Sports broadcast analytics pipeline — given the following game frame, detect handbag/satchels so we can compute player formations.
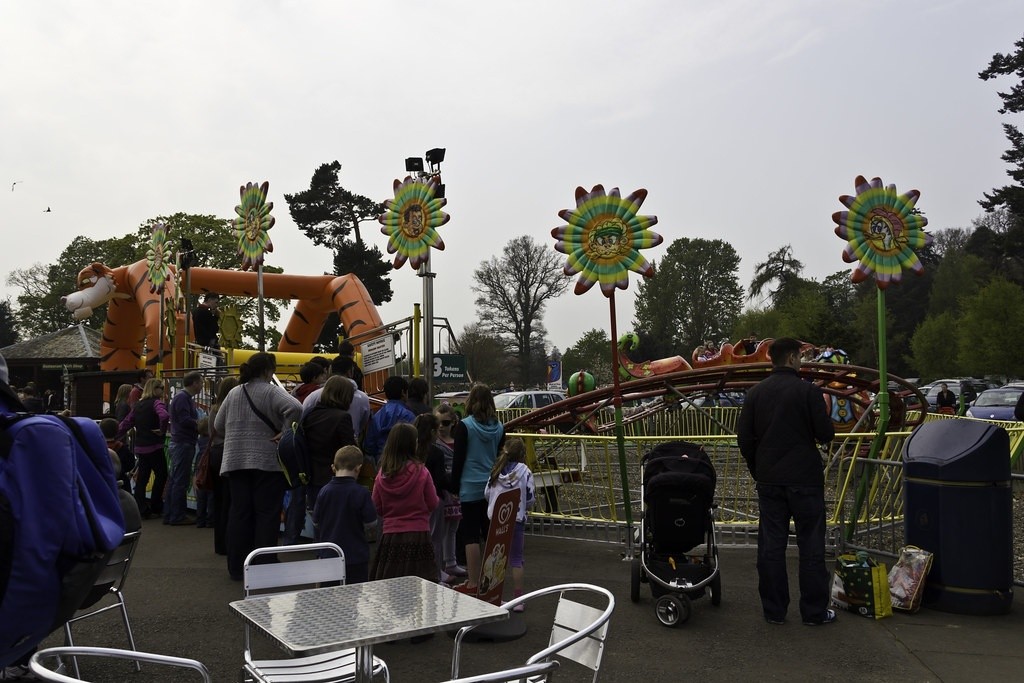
[832,554,893,619]
[886,545,934,613]
[194,445,209,491]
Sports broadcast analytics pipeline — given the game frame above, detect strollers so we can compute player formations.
[631,442,722,628]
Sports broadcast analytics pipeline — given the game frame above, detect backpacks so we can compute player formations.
[0,416,126,665]
[276,407,310,488]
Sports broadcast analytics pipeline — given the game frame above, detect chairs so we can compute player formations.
[233,542,393,683]
[451,581,618,683]
[27,645,214,683]
[62,526,144,679]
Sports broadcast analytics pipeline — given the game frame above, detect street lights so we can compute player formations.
[404,147,448,411]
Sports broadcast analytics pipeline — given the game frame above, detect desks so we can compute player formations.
[227,574,512,683]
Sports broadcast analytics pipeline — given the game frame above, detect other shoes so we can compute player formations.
[804,610,835,626]
[445,564,466,575]
[441,570,456,582]
[141,512,159,519]
[411,633,434,644]
[170,515,195,525]
[453,580,480,596]
[384,641,397,644]
[765,614,785,625]
[512,604,523,612]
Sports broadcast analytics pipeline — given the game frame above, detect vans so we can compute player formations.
[493,391,569,418]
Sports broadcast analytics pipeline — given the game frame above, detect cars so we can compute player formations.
[889,377,1024,421]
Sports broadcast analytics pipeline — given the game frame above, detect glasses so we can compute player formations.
[155,386,164,390]
[441,420,457,427]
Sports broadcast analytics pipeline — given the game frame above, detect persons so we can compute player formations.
[0,340,537,680]
[738,337,837,626]
[697,331,833,384]
[193,291,225,373]
[1014,391,1024,422]
[935,383,956,410]
